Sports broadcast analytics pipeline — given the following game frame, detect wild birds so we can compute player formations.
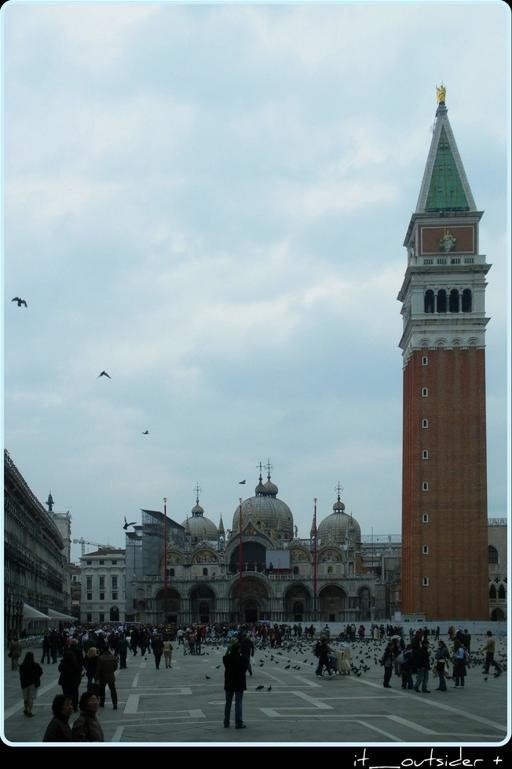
[99,370,111,379]
[142,430,149,434]
[238,480,246,484]
[255,685,264,691]
[200,638,507,682]
[11,296,28,308]
[267,685,272,691]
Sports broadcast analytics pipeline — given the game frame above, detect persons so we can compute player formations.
[6,621,503,742]
